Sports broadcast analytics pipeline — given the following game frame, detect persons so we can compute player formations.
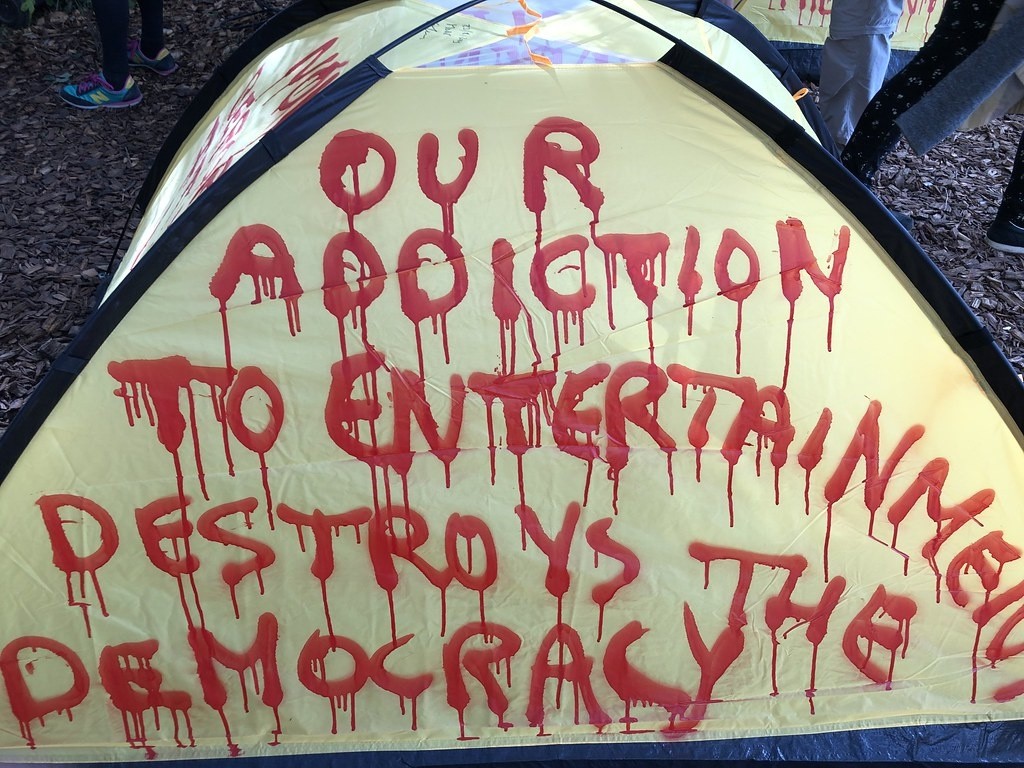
[58,1,181,110]
[834,2,1024,254]
[815,1,903,169]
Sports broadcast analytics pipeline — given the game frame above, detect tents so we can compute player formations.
[706,0,1011,136]
[0,0,1024,768]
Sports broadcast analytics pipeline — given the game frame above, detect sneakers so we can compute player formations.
[984,218,1024,256]
[126,42,179,76]
[60,70,144,109]
